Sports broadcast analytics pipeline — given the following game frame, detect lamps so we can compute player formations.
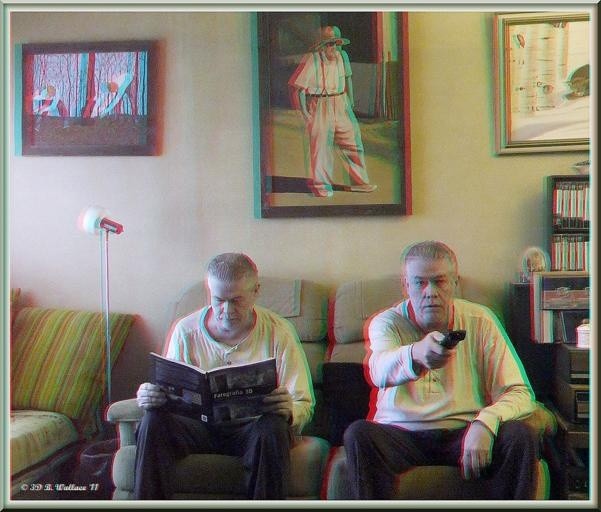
[82,204,130,408]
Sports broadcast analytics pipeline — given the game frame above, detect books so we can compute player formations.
[552,233,587,271]
[554,182,588,227]
[542,309,577,342]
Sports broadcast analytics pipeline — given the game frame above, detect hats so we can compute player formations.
[309,25,350,52]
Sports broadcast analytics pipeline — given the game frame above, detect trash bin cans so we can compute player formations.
[80,440,119,496]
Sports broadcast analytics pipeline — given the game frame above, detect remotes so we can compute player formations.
[438,330,468,350]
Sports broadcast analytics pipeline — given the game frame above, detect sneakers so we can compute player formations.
[344,185,378,192]
[315,191,335,197]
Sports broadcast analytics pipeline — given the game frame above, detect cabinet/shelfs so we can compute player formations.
[554,343,589,424]
[543,175,591,273]
[529,268,591,347]
[549,402,591,501]
[508,279,554,410]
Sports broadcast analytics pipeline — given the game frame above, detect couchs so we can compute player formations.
[321,270,556,501]
[104,275,332,499]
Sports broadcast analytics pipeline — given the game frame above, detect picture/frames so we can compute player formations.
[21,38,162,157]
[254,12,416,220]
[490,12,592,157]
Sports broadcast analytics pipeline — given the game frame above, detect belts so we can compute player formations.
[307,92,344,97]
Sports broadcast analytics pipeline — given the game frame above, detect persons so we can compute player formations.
[129,252,316,500]
[344,241,538,500]
[293,26,378,198]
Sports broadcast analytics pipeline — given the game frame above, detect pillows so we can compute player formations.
[9,286,21,324]
[10,304,139,422]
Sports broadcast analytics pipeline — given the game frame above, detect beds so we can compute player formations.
[9,294,143,499]
